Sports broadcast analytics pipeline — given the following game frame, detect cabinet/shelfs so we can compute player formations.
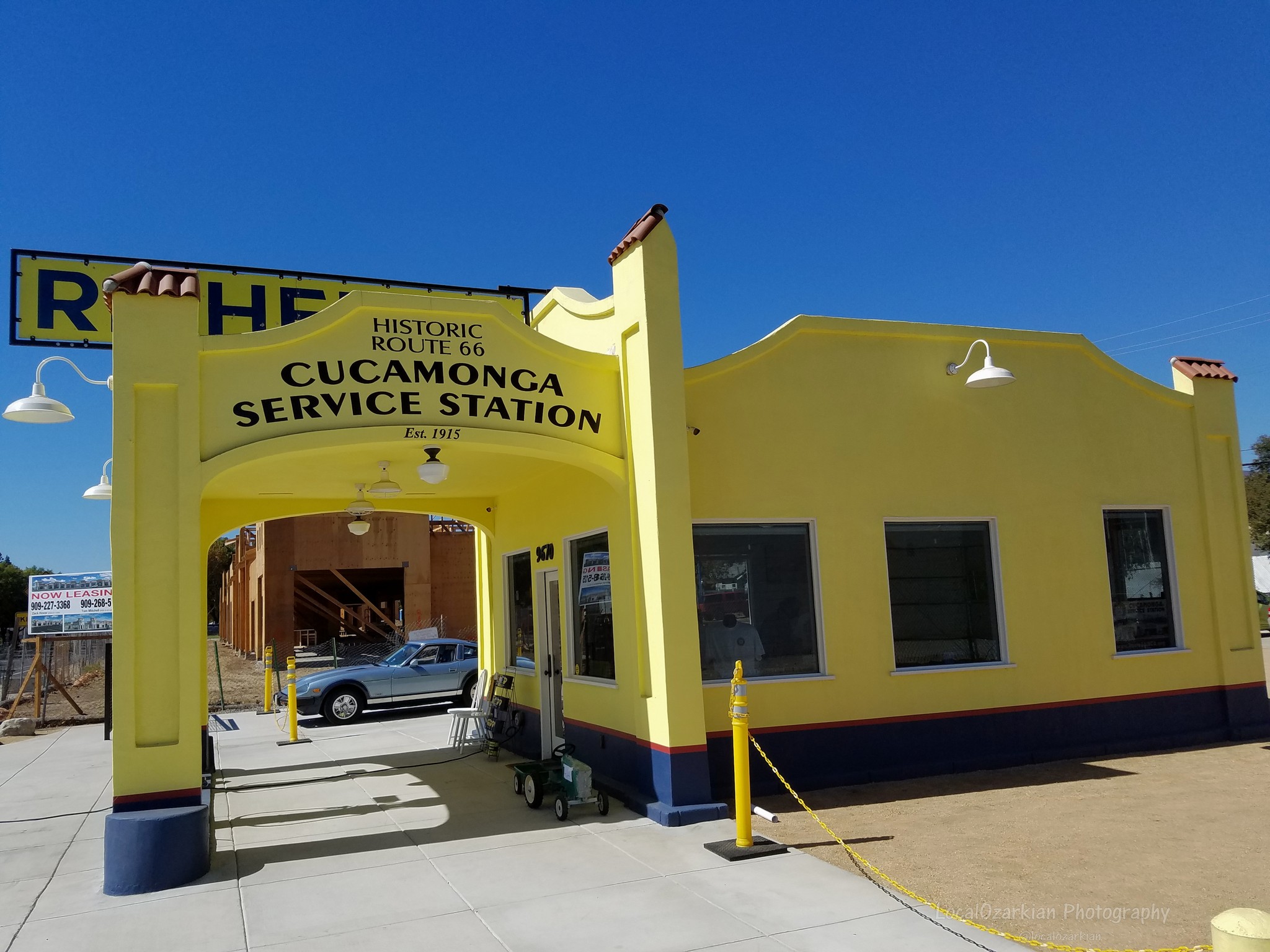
[479,665,516,762]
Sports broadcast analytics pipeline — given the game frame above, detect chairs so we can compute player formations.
[447,669,497,755]
[438,649,455,662]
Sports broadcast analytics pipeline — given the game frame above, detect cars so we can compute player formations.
[1250,543,1270,631]
[274,638,536,726]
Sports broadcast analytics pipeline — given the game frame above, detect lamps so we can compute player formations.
[1,356,114,424]
[416,443,449,485]
[82,459,112,501]
[947,340,1017,389]
[366,461,403,499]
[344,482,375,517]
[347,512,371,536]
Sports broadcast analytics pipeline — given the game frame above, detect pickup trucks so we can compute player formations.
[207,623,219,635]
